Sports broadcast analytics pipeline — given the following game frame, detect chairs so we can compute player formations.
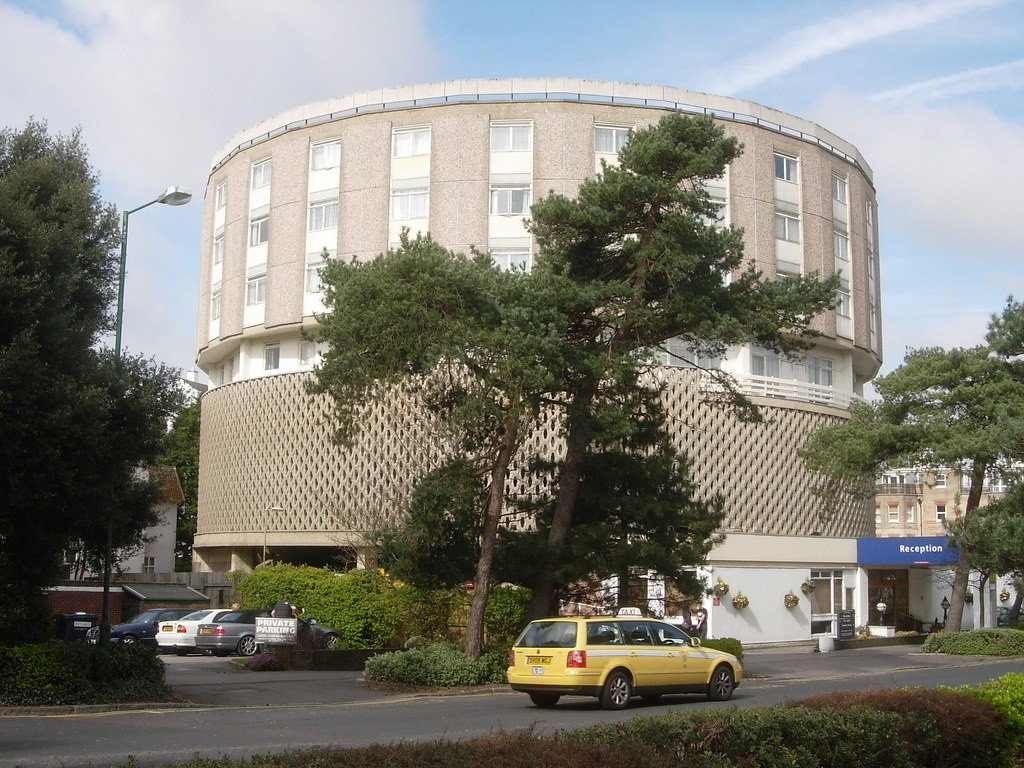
[633,629,650,644]
[602,631,615,644]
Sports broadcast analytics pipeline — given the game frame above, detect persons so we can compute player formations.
[271,597,296,618]
[696,602,707,637]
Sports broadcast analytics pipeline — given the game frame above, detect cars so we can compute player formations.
[194,608,342,656]
[86,608,203,655]
[154,608,235,656]
[506,607,743,710]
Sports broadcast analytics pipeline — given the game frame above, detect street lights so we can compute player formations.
[263,507,284,567]
[98,186,193,647]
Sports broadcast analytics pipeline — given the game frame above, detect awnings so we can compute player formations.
[123,582,210,602]
